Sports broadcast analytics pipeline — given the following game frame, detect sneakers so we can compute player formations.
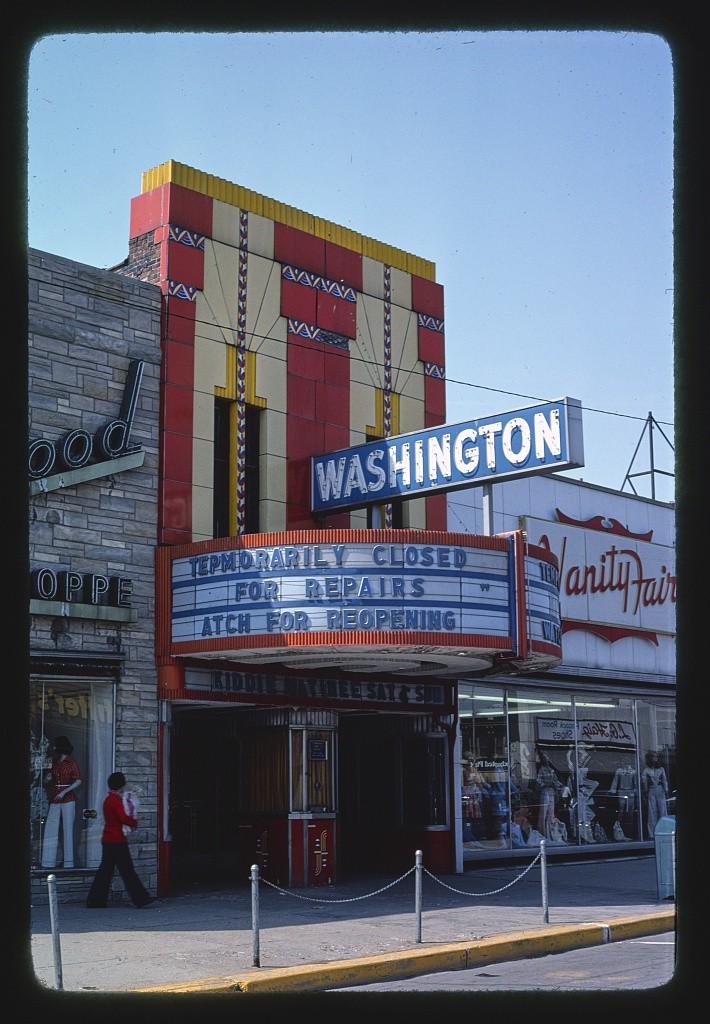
[85,901,110,908]
[137,899,162,908]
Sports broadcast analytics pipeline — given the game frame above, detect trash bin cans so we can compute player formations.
[654,814,676,901]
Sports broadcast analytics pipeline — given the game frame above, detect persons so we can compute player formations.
[39,736,83,870]
[609,753,646,842]
[85,771,161,909]
[534,750,570,839]
[461,750,491,842]
[640,750,671,839]
[487,760,522,839]
